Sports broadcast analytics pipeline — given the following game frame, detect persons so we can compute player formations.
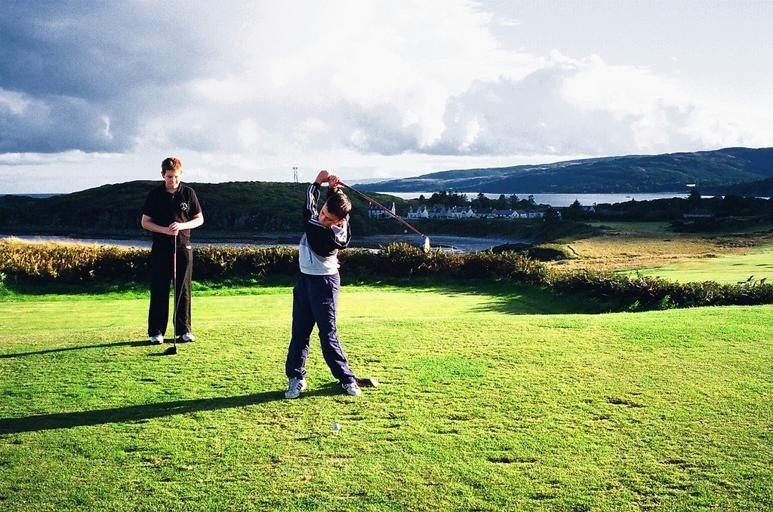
[139,156,205,345]
[282,170,366,399]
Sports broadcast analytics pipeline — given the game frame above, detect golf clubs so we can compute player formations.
[163,235,177,354]
[327,176,431,254]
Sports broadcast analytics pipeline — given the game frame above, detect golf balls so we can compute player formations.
[333,423,340,430]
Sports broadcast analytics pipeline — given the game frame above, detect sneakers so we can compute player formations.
[285,377,307,399]
[342,382,362,396]
[151,334,164,343]
[182,333,195,342]
